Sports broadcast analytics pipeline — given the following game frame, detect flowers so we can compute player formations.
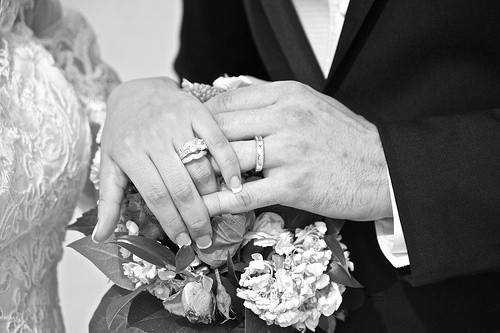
[65,177,363,333]
